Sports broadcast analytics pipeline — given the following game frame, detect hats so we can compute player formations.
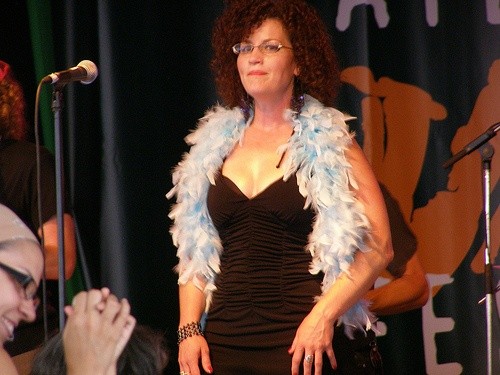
[0,202,40,246]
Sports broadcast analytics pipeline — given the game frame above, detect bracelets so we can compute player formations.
[176,322,205,345]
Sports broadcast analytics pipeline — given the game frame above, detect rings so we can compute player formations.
[304,354,313,363]
[180,371,190,375]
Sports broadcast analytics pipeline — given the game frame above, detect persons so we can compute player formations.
[165,0,395,375]
[363,174,430,315]
[0,60,171,375]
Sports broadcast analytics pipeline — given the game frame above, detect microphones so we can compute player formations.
[42,60,98,84]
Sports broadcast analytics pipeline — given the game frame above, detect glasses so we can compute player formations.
[0,261,40,308]
[232,40,292,56]
